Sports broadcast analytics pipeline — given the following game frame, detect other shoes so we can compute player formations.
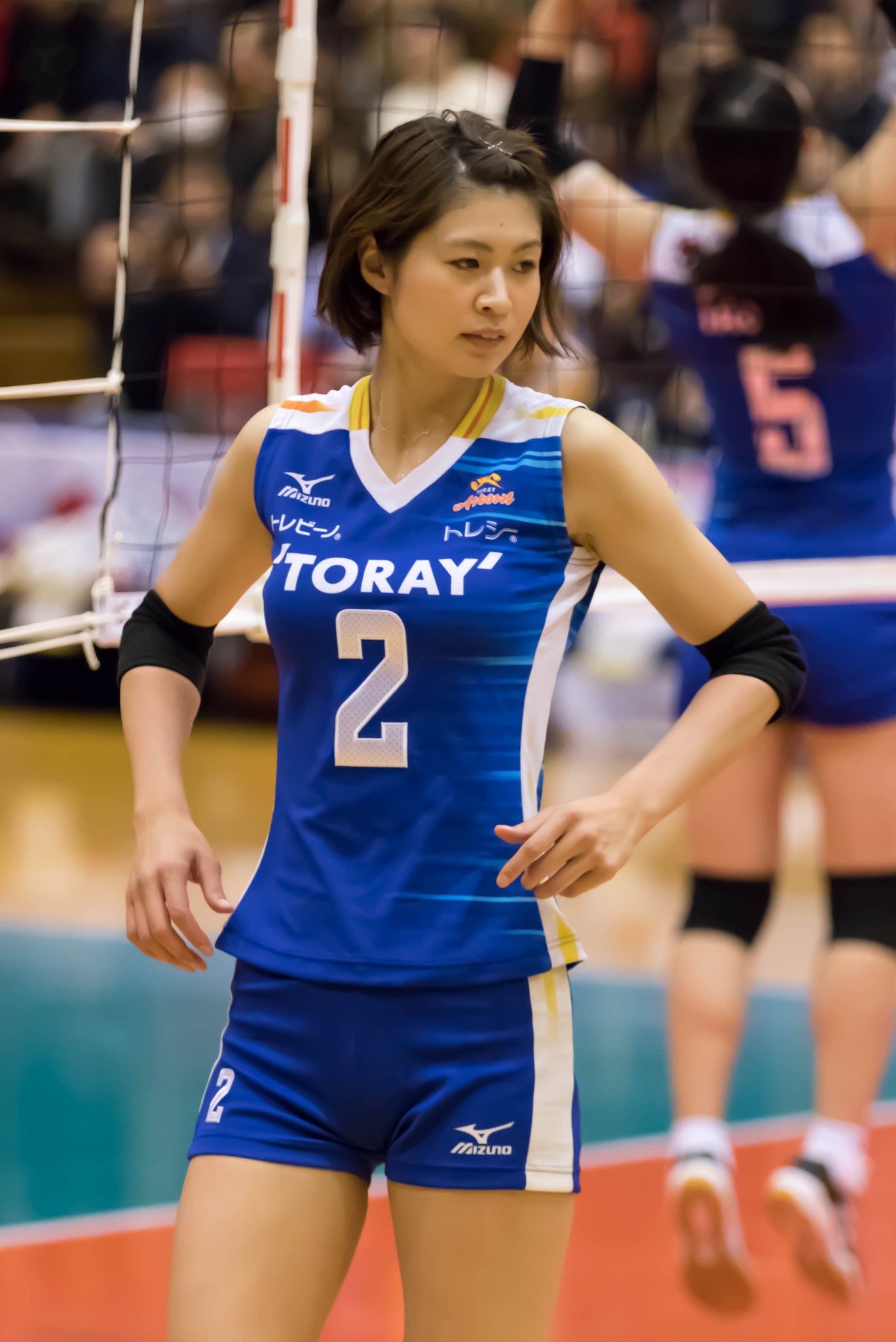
[763,1156,861,1303]
[665,1151,756,1318]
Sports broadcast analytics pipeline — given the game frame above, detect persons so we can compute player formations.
[0,1,894,1317]
[114,112,796,1342]
